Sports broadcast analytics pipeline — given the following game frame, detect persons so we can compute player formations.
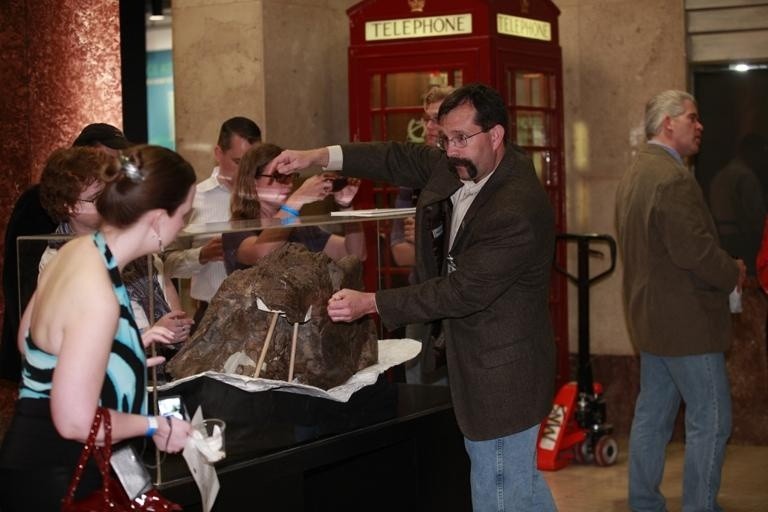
[38,142,197,385]
[755,214,768,296]
[709,133,765,254]
[0,122,129,383]
[612,88,748,512]
[275,30,561,511]
[164,115,263,336]
[388,84,458,385]
[221,140,370,275]
[0,145,196,512]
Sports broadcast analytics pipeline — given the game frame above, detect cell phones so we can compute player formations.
[157,395,183,419]
[326,176,349,192]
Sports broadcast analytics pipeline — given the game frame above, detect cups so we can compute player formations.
[192,418,227,464]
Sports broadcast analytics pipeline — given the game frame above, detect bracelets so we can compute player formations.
[279,204,300,217]
[145,414,159,436]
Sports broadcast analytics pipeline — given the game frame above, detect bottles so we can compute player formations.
[728,285,743,314]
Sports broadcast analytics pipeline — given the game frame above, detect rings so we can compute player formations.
[178,320,182,326]
[181,325,185,331]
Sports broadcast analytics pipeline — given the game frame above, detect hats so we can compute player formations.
[81,123,129,149]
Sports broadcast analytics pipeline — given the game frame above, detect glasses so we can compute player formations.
[434,131,485,150]
[76,192,98,206]
[261,171,296,185]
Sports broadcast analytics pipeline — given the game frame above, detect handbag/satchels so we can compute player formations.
[63,407,182,510]
